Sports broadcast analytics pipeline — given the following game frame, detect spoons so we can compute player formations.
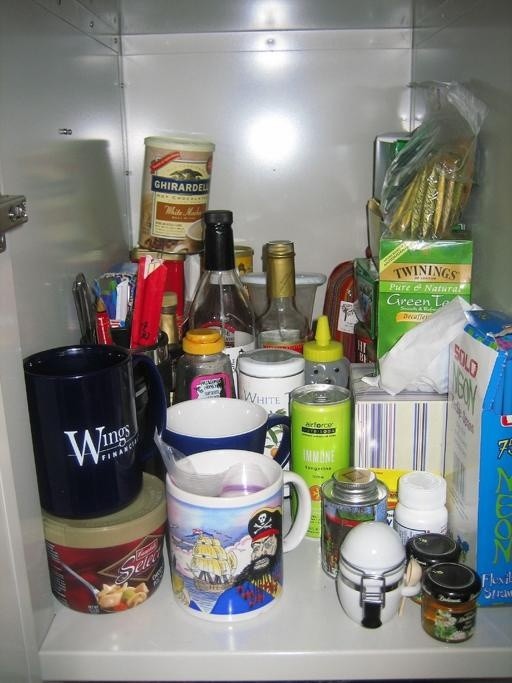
[396,557,422,617]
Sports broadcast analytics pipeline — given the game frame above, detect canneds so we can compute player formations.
[406,533,460,605]
[420,563,481,643]
[320,466,388,580]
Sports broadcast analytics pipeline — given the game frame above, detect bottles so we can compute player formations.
[190,210,254,332]
[289,383,351,540]
[303,315,350,389]
[138,136,216,255]
[393,470,448,547]
[258,241,310,349]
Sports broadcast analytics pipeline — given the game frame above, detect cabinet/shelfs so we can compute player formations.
[0,1,512,682]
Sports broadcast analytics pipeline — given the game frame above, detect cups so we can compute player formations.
[23,345,167,519]
[240,271,328,329]
[166,399,291,470]
[166,449,312,622]
